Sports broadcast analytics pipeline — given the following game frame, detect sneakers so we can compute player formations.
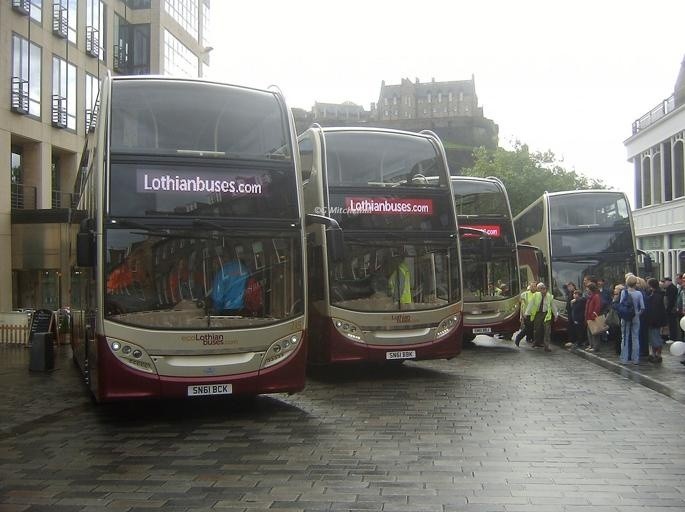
[526,337,532,343]
[618,358,628,365]
[544,348,552,352]
[565,340,590,349]
[654,356,663,363]
[648,355,658,364]
[632,361,640,367]
[515,336,520,347]
[665,339,677,344]
[589,347,600,352]
[534,342,545,348]
[584,347,595,352]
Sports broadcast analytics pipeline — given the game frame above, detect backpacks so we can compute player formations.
[242,270,265,311]
[615,290,636,321]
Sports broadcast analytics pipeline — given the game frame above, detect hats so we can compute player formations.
[661,277,672,282]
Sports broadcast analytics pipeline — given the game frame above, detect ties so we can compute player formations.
[538,295,546,312]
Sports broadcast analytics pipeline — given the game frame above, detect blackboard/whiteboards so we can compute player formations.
[27,309,53,344]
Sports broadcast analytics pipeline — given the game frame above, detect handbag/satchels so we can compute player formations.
[570,319,586,340]
[604,308,621,328]
[586,312,610,336]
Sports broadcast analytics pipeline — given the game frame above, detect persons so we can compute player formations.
[515,269,685,364]
